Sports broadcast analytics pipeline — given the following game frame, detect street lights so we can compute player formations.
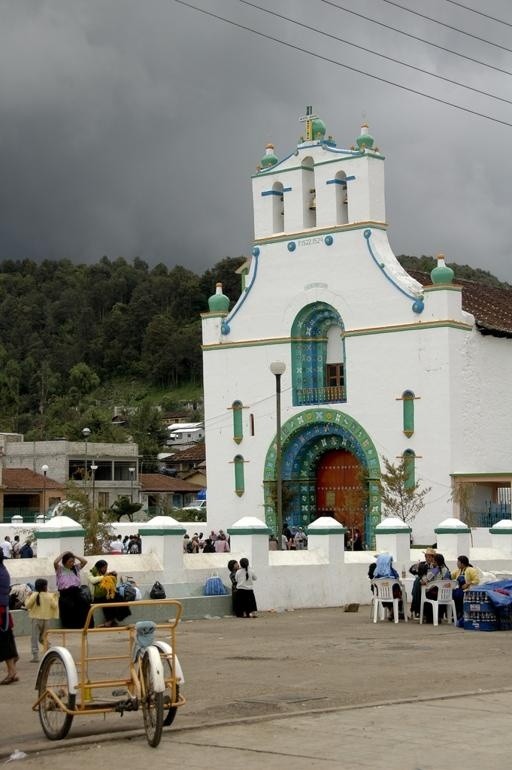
[268,359,287,552]
[36,426,136,536]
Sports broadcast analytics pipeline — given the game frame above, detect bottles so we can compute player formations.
[401,563,406,578]
[467,592,512,624]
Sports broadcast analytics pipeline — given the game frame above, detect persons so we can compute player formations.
[88,560,131,625]
[229,559,259,617]
[343,528,362,550]
[367,548,478,624]
[184,530,230,553]
[106,533,142,555]
[277,522,307,551]
[0,524,34,560]
[24,579,59,663]
[0,549,20,682]
[53,551,95,629]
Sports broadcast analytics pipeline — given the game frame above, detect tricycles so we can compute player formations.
[32,597,185,751]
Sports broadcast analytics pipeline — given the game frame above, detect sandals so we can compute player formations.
[1,673,18,684]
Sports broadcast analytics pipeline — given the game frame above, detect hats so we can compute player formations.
[422,548,436,555]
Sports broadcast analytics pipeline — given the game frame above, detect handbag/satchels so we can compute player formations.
[118,577,142,601]
[204,571,228,595]
[150,581,166,599]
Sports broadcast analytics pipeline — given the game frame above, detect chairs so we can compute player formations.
[371,577,410,623]
[419,580,458,626]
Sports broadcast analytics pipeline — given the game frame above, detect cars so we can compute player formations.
[181,499,206,510]
